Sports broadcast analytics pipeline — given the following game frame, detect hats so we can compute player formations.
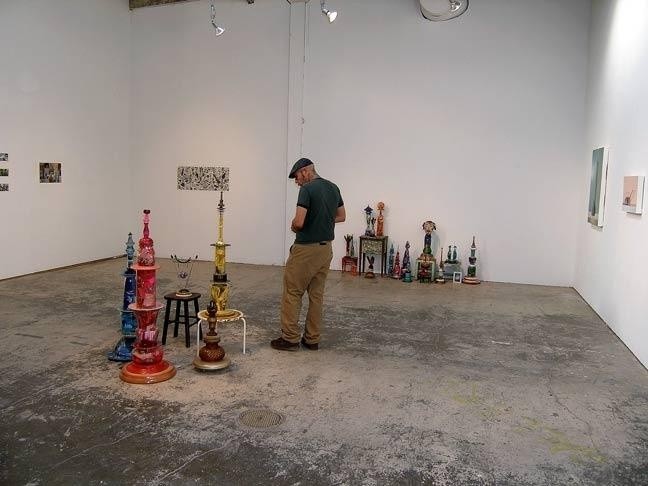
[288,158,313,179]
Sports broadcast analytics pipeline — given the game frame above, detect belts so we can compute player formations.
[320,243,326,245]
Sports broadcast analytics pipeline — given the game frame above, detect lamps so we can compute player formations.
[319,1,339,22]
[205,0,226,39]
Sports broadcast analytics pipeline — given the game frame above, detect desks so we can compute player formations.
[358,234,389,279]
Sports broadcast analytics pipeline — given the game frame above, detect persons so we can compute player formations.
[269,157,347,353]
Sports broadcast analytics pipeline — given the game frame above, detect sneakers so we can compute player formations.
[270,336,318,351]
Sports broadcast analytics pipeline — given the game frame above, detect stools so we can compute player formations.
[161,290,206,348]
[341,256,357,276]
[194,307,247,359]
[415,259,435,282]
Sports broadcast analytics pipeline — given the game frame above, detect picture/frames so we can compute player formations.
[586,146,609,230]
[619,173,644,216]
[452,271,462,284]
